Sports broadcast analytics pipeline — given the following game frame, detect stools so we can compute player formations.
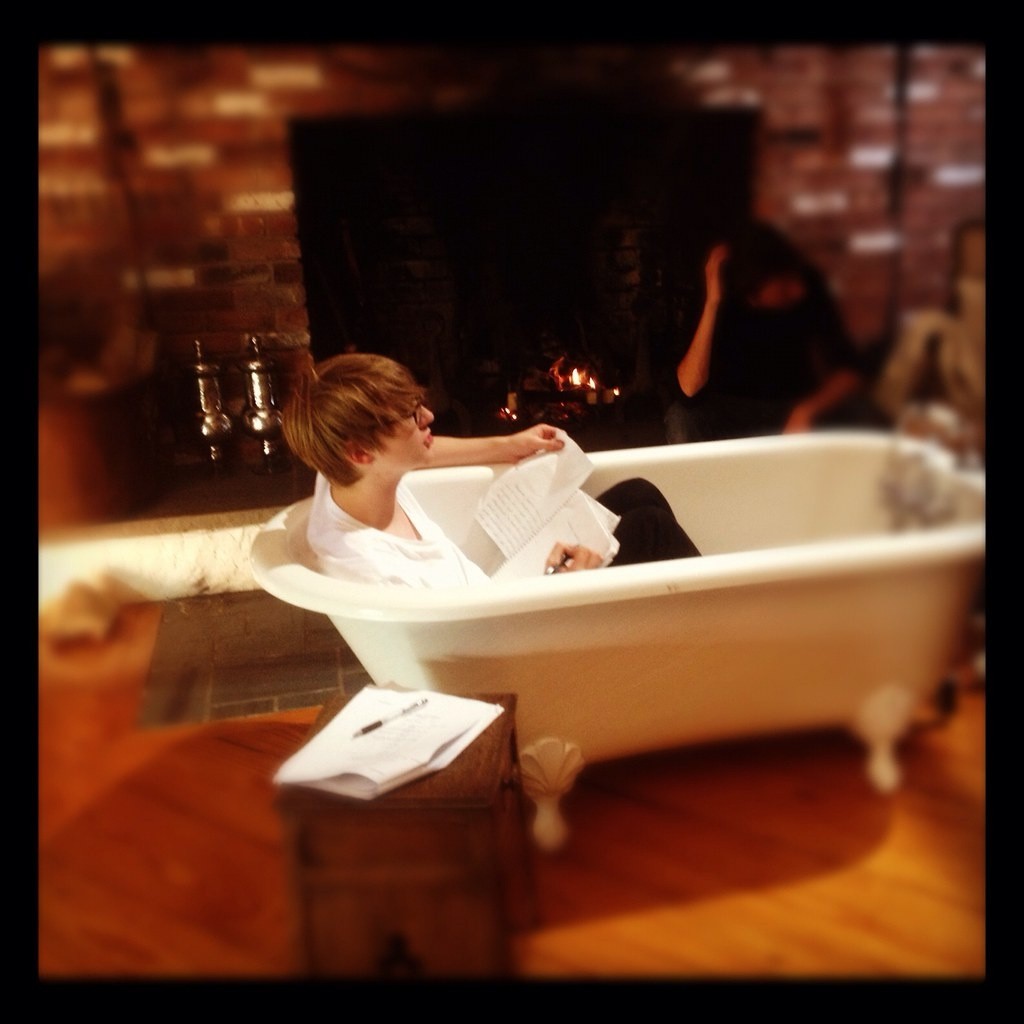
[292,688,532,981]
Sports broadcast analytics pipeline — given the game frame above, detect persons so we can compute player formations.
[281,355,700,588]
[649,219,985,470]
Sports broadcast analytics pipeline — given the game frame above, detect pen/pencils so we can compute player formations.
[545,543,581,575]
[353,698,428,739]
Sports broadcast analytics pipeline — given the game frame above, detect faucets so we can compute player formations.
[903,401,985,472]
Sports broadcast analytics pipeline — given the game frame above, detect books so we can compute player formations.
[488,488,622,581]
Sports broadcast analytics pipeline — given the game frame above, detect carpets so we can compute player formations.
[137,586,344,735]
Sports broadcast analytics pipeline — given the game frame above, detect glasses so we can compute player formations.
[369,399,423,430]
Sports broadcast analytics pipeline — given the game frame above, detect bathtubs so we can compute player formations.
[242,426,985,793]
